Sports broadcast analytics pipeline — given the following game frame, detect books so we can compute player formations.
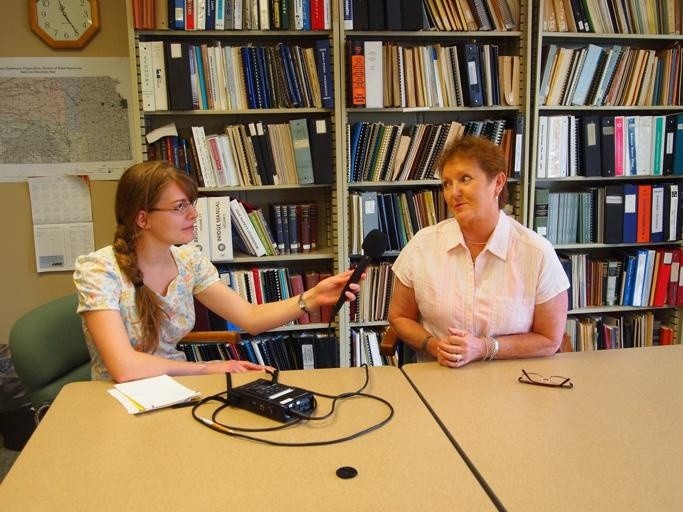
[350,185,515,255]
[349,260,394,323]
[351,327,437,368]
[132,1,337,371]
[533,1,682,353]
[345,35,520,108]
[108,374,203,415]
[346,117,524,183]
[343,0,521,32]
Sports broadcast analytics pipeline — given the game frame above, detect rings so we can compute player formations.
[456,353,464,362]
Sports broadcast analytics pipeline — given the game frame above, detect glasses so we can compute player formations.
[147,199,196,214]
[519,369,573,388]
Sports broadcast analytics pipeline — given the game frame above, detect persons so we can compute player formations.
[73,159,368,384]
[389,134,571,368]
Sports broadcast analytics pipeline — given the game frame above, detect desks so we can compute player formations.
[402,335,680,511]
[2,359,505,511]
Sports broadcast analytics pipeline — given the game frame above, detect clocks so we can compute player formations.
[26,0,103,50]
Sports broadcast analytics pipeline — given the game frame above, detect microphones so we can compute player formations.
[328,229,388,319]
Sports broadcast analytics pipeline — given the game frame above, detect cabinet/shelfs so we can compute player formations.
[335,0,534,370]
[121,0,344,374]
[533,1,681,353]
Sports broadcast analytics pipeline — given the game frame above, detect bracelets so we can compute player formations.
[481,336,499,361]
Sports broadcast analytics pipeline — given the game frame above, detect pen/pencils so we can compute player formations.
[172,400,199,409]
[201,418,232,434]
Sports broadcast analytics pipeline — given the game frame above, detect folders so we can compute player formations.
[653,248,683,305]
[464,43,483,107]
[164,41,194,111]
[289,112,334,185]
[362,191,379,241]
[605,185,637,244]
[581,115,615,178]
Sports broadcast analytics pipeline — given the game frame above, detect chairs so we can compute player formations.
[8,292,240,428]
[377,313,571,366]
